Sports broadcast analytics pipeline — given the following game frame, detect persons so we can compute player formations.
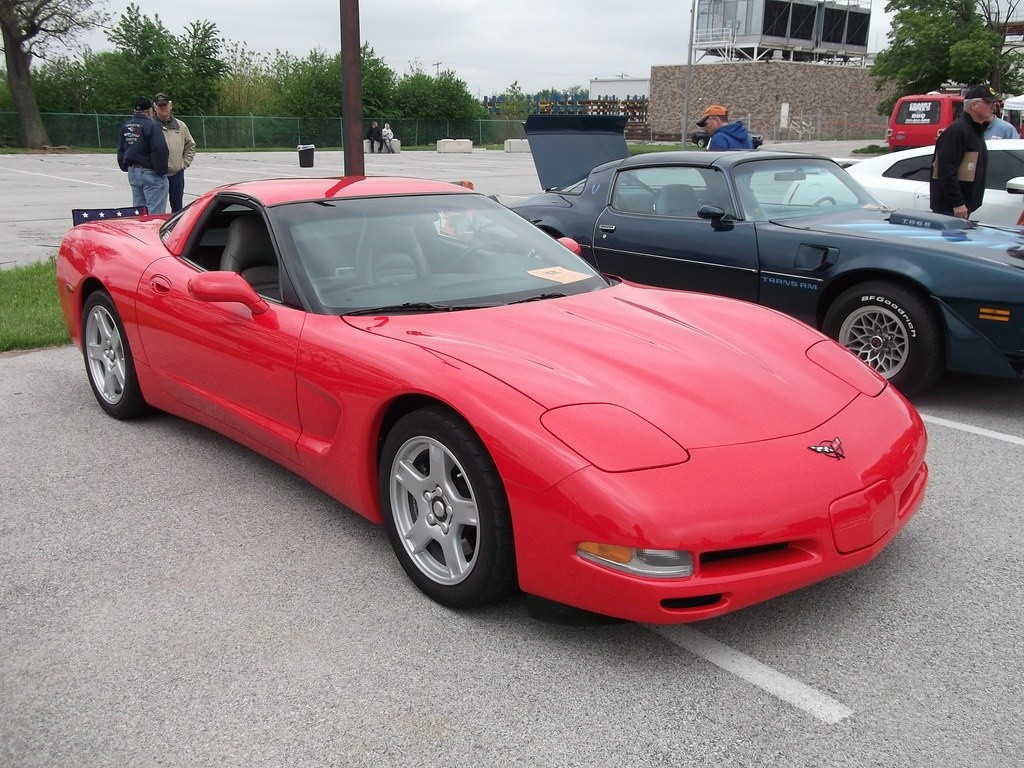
[117,96,169,214]
[696,105,753,185]
[929,85,1002,219]
[382,123,395,153]
[983,114,1018,140]
[367,122,384,152]
[150,94,196,213]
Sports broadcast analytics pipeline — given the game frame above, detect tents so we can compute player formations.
[1001,94,1024,120]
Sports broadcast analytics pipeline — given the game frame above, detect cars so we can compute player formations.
[782,139,1024,239]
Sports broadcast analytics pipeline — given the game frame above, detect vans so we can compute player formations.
[886,88,964,152]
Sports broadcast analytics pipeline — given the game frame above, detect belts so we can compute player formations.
[130,163,145,168]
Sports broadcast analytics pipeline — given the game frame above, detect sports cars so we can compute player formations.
[55,175,929,627]
[496,151,1024,401]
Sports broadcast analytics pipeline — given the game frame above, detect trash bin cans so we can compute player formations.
[298,145,315,168]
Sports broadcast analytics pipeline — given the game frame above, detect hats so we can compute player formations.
[964,84,1002,103]
[154,93,169,106]
[134,97,154,112]
[696,105,728,127]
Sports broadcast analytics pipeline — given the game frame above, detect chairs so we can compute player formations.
[219,212,281,299]
[356,222,431,285]
[720,183,766,217]
[651,183,699,216]
